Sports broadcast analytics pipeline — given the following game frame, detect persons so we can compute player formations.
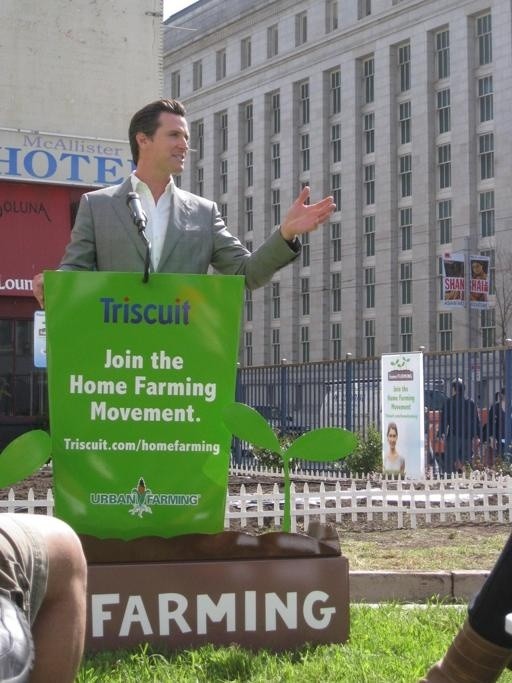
[33,98,336,310]
[472,261,487,278]
[384,422,405,477]
[436,378,482,475]
[1,512,88,682]
[487,387,505,448]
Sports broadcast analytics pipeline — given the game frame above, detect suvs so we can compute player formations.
[240,399,313,460]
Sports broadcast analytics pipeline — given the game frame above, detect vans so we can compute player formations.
[317,375,494,468]
